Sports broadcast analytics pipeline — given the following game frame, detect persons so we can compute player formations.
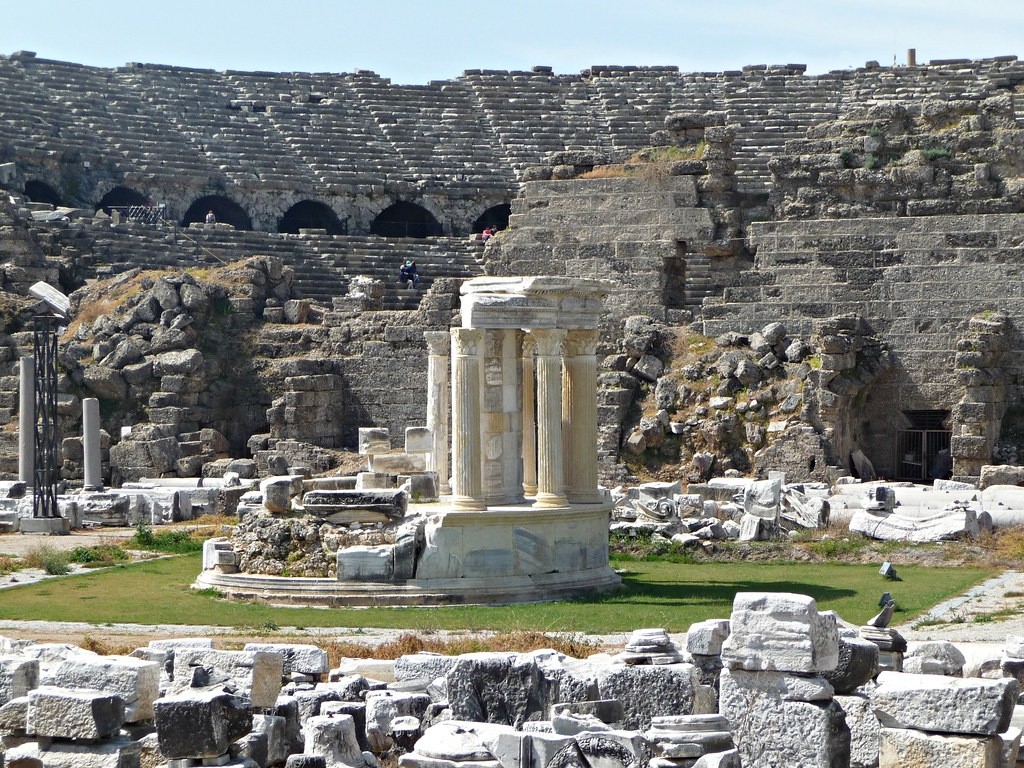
[206,210,216,223]
[489,224,498,236]
[398,257,422,292]
[482,227,492,241]
